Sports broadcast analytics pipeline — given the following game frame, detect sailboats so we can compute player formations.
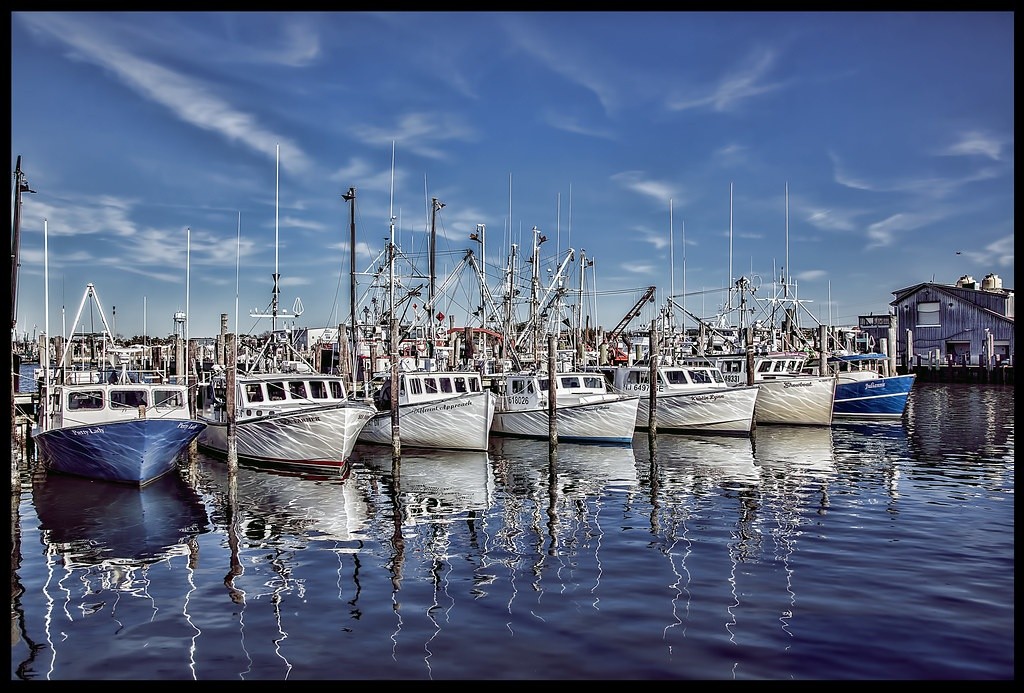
[31,462,213,680]
[26,138,918,487]
[26,214,208,491]
[193,413,917,660]
